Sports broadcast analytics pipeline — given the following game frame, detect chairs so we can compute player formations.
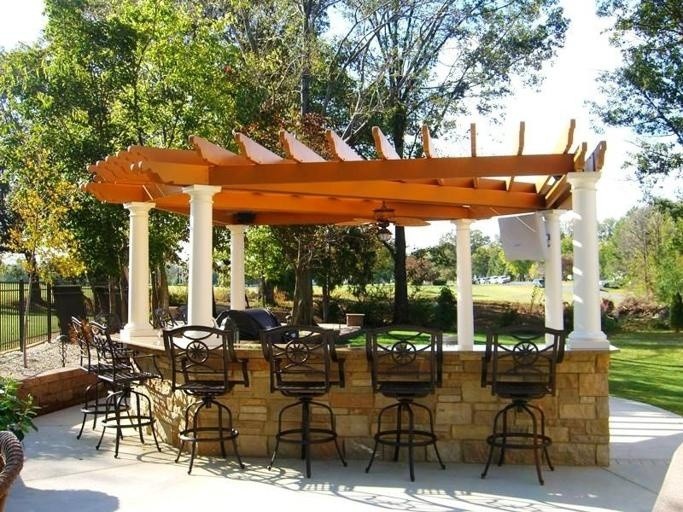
[0,430,24,512]
[154,307,178,328]
[259,322,347,477]
[69,315,122,438]
[362,317,444,481]
[162,325,248,475]
[477,318,566,485]
[85,320,161,459]
